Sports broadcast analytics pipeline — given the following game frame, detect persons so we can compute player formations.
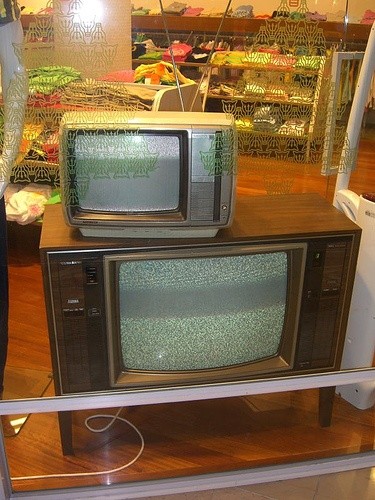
[0,1,29,400]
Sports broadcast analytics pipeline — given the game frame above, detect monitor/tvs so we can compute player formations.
[37,190,363,456]
[58,110,238,238]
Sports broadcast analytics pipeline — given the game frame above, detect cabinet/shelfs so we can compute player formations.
[0,84,204,228]
[132,54,331,159]
